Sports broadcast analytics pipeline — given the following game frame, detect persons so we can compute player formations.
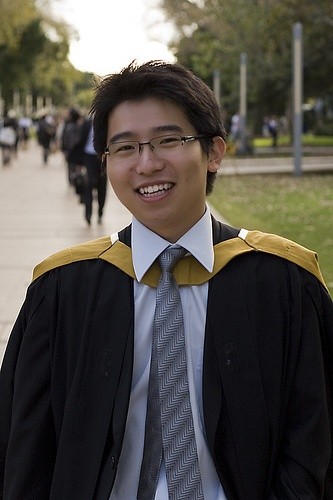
[0,98,332,226]
[0,57,333,500]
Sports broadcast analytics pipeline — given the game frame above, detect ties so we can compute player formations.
[137,246,207,500]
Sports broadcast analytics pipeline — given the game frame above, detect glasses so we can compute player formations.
[102,134,205,162]
